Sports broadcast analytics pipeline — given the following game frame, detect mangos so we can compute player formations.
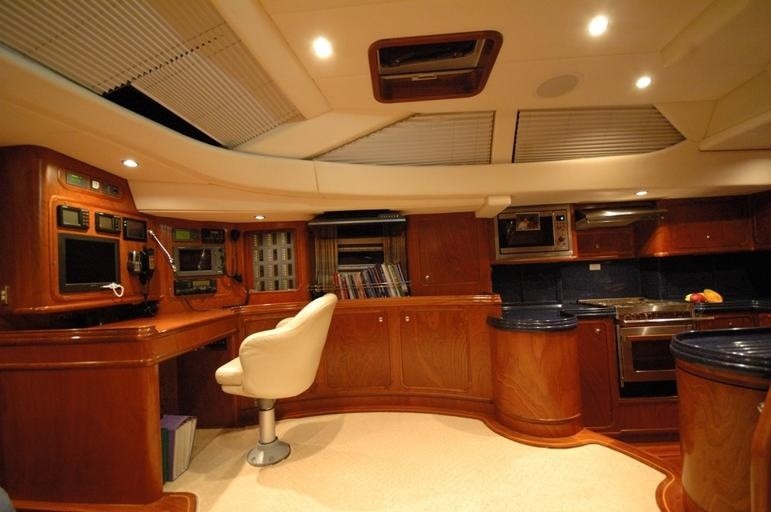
[703,289,723,303]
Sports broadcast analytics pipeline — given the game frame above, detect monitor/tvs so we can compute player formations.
[57,233,120,292]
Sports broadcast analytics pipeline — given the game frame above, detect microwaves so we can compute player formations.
[496,204,575,261]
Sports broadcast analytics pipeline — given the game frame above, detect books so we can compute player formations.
[335,263,409,299]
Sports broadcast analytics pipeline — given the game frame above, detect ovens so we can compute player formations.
[618,312,692,397]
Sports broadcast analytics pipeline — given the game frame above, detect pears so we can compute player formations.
[684,294,692,302]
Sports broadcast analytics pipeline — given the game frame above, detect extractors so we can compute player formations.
[575,207,668,227]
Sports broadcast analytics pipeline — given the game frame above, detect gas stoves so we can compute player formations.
[577,297,689,310]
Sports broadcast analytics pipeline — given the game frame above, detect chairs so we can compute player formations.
[214,293,340,468]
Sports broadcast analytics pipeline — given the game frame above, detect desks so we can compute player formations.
[2,305,242,511]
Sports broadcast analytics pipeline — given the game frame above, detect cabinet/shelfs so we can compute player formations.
[659,199,755,254]
[322,297,502,420]
[691,316,756,332]
[236,314,321,421]
[577,226,635,260]
[406,212,494,295]
[615,322,696,443]
[575,318,619,437]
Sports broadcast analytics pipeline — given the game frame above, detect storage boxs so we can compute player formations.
[161,412,198,482]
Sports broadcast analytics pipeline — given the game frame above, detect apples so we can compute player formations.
[691,294,706,303]
